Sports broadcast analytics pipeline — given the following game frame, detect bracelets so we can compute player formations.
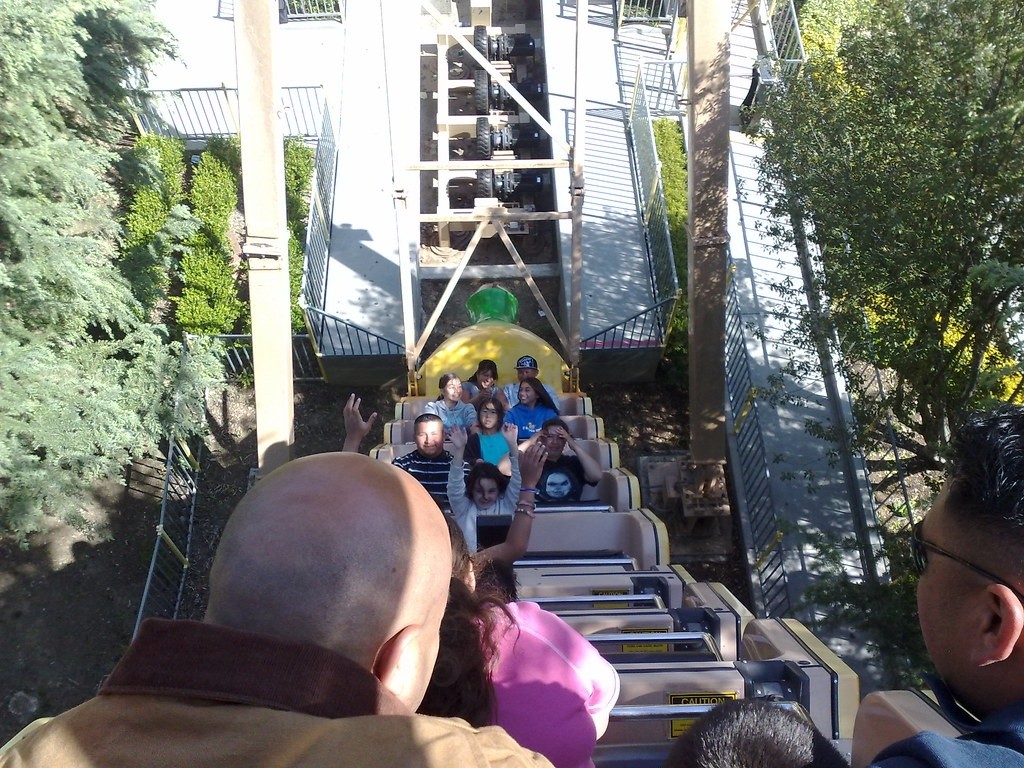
[515,508,534,518]
[519,488,540,494]
[516,501,536,509]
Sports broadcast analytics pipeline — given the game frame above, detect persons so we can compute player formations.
[866,415,1024,768]
[503,355,560,409]
[662,698,852,768]
[342,394,621,768]
[424,373,479,439]
[461,359,509,411]
[0,451,557,768]
[503,378,560,440]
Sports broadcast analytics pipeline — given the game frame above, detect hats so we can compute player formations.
[514,355,537,370]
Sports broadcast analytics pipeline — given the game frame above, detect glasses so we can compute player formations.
[912,520,1024,605]
